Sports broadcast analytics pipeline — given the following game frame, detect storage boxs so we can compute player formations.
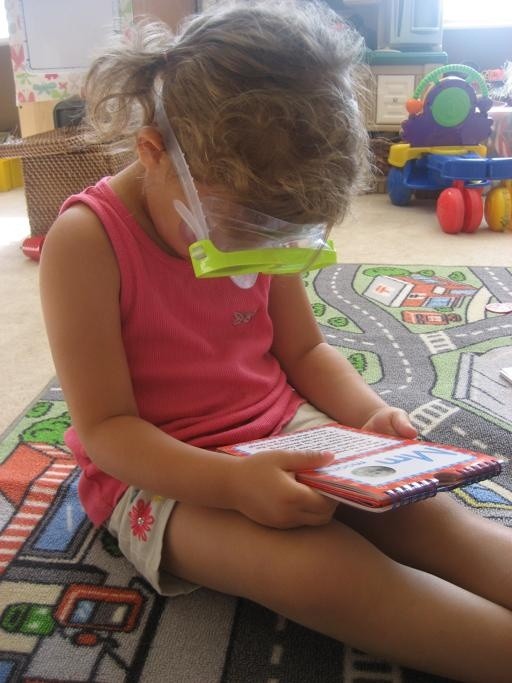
[2,124,140,239]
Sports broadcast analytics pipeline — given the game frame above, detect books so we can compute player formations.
[213,420,498,516]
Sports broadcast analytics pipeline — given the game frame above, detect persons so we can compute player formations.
[38,0,512,682]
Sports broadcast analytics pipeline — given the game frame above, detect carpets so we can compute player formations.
[0,262,509,679]
